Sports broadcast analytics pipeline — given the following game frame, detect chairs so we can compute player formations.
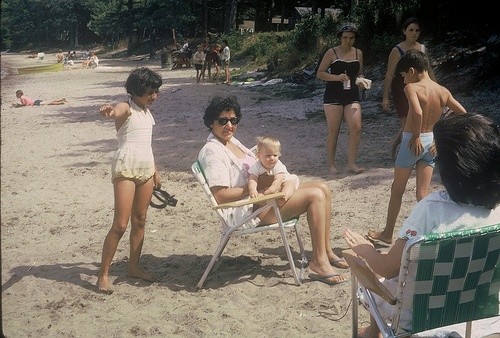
[337,225,499,337]
[192,160,308,292]
[171,54,191,70]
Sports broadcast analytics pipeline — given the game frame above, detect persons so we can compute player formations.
[315,21,364,174]
[382,20,435,160]
[197,93,353,286]
[249,135,302,208]
[22,43,106,70]
[340,108,500,338]
[13,90,66,107]
[96,68,163,296]
[160,23,240,84]
[365,49,469,244]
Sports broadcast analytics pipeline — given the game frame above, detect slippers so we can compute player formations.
[309,273,347,284]
[330,257,350,269]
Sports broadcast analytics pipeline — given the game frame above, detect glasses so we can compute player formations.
[341,25,358,32]
[215,118,240,126]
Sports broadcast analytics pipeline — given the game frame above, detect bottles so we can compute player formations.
[343,80,351,90]
